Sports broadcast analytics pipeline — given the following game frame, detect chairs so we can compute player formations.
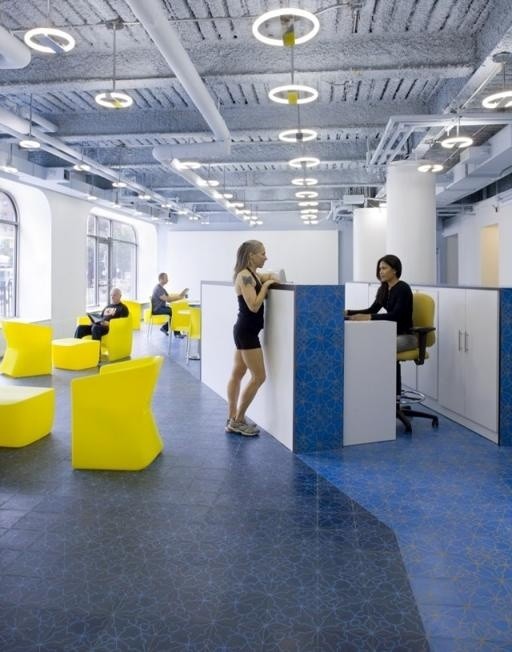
[0,294,202,470]
[395,292,439,432]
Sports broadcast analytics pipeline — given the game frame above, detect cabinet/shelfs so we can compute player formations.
[345,282,500,431]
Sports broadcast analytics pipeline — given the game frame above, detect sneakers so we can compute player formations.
[225,419,260,435]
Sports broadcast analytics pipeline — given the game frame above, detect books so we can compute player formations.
[86,312,111,324]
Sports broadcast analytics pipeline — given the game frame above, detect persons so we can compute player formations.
[151,273,185,339]
[343,255,417,353]
[225,240,280,436]
[74,288,129,368]
